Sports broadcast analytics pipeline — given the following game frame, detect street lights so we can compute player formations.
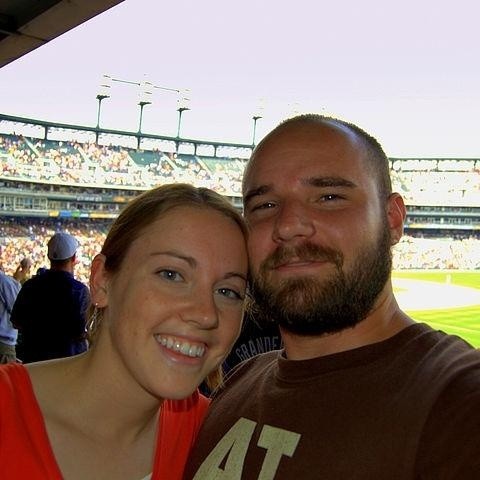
[135,101,150,148]
[174,107,191,154]
[250,115,262,149]
[95,94,110,146]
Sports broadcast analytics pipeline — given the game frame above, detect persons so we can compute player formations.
[1,129,480,290]
[0,180,252,480]
[197,310,284,398]
[0,261,23,364]
[11,233,92,363]
[182,112,480,478]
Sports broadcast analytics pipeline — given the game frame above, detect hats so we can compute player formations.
[48,232,81,260]
